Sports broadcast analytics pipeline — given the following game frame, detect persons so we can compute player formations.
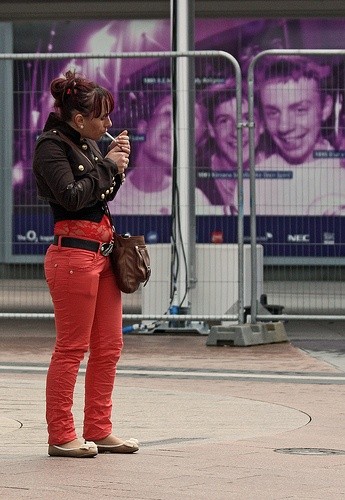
[194,77,274,206]
[233,55,345,216]
[34,71,137,456]
[108,93,213,206]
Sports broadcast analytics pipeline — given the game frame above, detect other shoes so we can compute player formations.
[86,437,138,455]
[48,439,98,458]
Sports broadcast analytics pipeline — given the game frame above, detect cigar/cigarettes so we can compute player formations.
[105,132,116,142]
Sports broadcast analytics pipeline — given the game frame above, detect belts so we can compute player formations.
[52,234,113,254]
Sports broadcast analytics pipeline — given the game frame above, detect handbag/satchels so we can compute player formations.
[110,230,152,293]
